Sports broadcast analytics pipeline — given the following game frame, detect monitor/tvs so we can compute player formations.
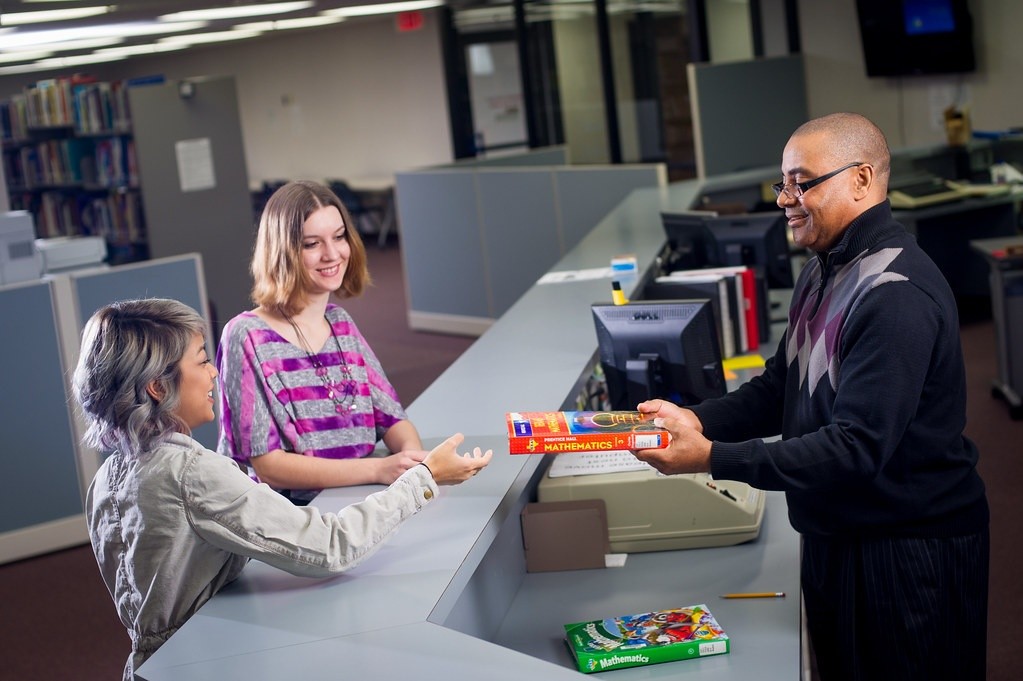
[855,0,978,80]
[590,211,793,412]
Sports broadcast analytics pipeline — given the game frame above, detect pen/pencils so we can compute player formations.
[719,592,786,598]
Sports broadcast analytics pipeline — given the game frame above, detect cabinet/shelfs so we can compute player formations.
[0,124,149,265]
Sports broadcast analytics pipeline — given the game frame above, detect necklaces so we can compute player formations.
[278,303,359,416]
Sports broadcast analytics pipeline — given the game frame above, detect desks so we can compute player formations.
[971,239,1023,415]
[489,242,809,679]
[887,161,1022,247]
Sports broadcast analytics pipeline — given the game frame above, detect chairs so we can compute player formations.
[260,178,385,244]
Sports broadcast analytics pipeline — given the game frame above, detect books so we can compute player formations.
[505,411,669,455]
[655,266,759,358]
[564,604,730,673]
[9,72,165,243]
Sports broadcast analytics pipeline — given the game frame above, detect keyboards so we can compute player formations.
[897,180,956,199]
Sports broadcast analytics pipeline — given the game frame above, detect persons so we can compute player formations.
[629,112,987,681]
[214,179,432,506]
[71,298,493,680]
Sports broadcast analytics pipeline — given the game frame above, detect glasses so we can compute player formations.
[771,162,870,198]
